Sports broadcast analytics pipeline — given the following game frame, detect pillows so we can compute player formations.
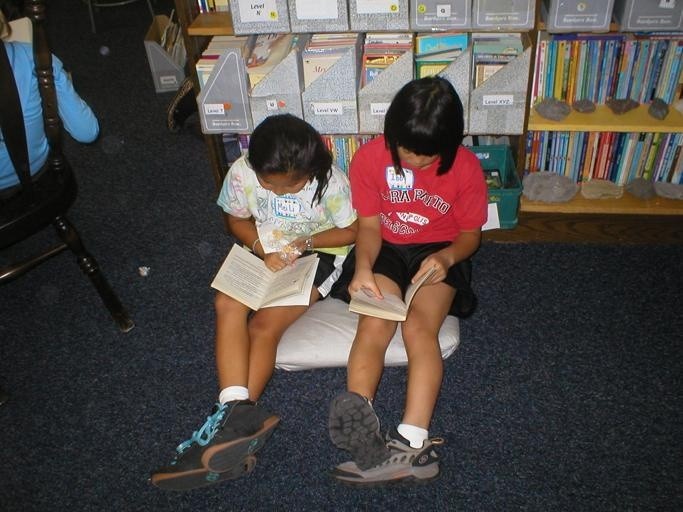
[272,291,464,374]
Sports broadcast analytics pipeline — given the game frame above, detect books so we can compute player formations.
[349,263,436,322]
[211,243,321,312]
[197,1,683,185]
[145,16,185,92]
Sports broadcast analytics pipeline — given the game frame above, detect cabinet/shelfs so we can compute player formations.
[180,0,537,257]
[519,0,682,223]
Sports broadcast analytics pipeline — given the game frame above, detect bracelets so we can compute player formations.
[303,234,315,253]
[252,239,259,253]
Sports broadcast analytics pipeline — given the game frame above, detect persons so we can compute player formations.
[152,114,361,490]
[326,76,486,483]
[0,39,100,189]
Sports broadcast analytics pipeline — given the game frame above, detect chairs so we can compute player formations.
[0,1,136,338]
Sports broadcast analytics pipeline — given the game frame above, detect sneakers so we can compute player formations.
[198,399,282,474]
[325,391,391,471]
[149,431,260,494]
[325,425,443,487]
